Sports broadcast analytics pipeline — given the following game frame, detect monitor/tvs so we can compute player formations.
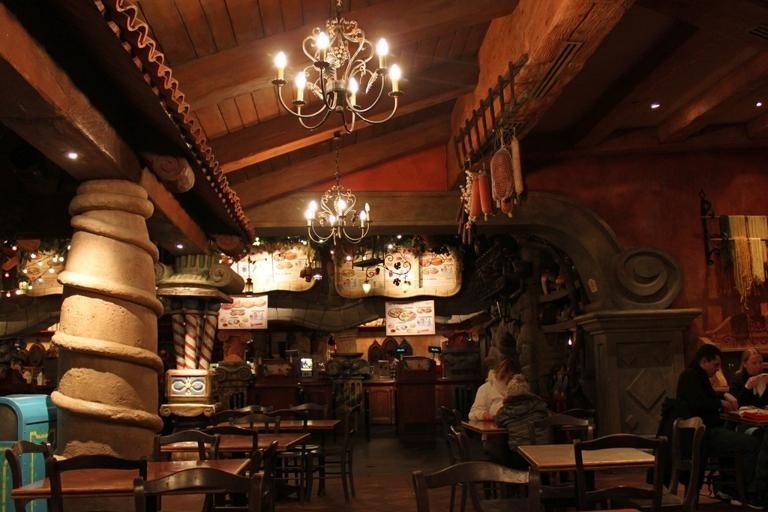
[285,350,298,360]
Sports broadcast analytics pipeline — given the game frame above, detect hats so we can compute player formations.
[506,373,530,397]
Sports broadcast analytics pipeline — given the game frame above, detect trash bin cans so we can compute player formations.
[0,393,57,512]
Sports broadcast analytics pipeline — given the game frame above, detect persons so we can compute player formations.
[728,346,768,409]
[380,350,399,379]
[494,376,555,495]
[468,355,523,490]
[672,345,765,510]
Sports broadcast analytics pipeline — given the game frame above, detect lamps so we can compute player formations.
[244,254,253,294]
[271,0,406,134]
[303,136,373,245]
